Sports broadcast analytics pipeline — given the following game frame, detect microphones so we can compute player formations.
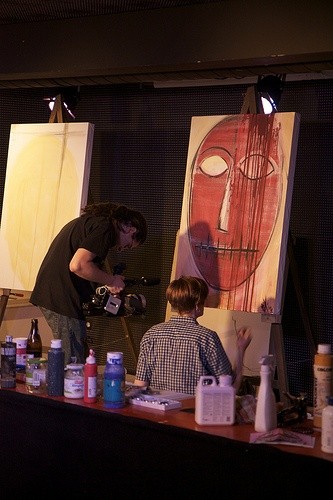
[141,278,160,286]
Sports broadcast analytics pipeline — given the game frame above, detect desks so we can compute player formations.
[0,374,333,462]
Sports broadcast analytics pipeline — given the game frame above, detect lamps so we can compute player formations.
[249,76,284,114]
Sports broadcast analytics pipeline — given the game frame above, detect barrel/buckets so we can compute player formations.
[196,376,236,426]
[12,338,27,371]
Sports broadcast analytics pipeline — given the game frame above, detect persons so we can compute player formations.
[136,276,253,395]
[29,202,146,365]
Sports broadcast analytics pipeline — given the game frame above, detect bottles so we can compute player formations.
[27,358,47,394]
[84,350,98,404]
[26,319,42,359]
[63,363,85,398]
[0,336,16,388]
[47,339,64,397]
[103,350,125,409]
[321,400,333,453]
[313,354,331,427]
